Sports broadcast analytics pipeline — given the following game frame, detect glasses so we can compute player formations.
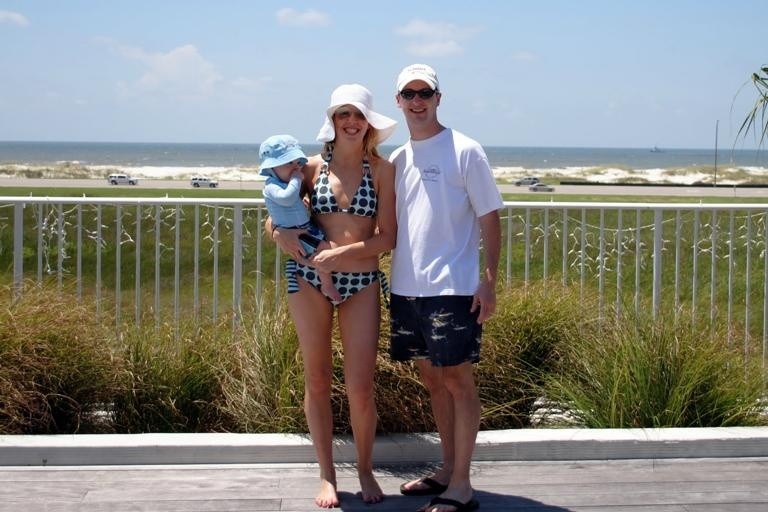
[401,90,433,99]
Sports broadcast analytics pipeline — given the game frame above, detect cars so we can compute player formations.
[108,173,138,185]
[190,176,219,188]
[513,176,555,192]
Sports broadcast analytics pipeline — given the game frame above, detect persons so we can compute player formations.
[263,83,397,511]
[387,64,507,512]
[255,134,342,303]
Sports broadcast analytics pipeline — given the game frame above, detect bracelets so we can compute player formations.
[271,227,277,239]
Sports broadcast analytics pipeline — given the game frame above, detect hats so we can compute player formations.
[395,63,439,91]
[316,84,397,144]
[258,135,308,176]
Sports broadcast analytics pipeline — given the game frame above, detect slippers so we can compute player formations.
[400,476,480,512]
